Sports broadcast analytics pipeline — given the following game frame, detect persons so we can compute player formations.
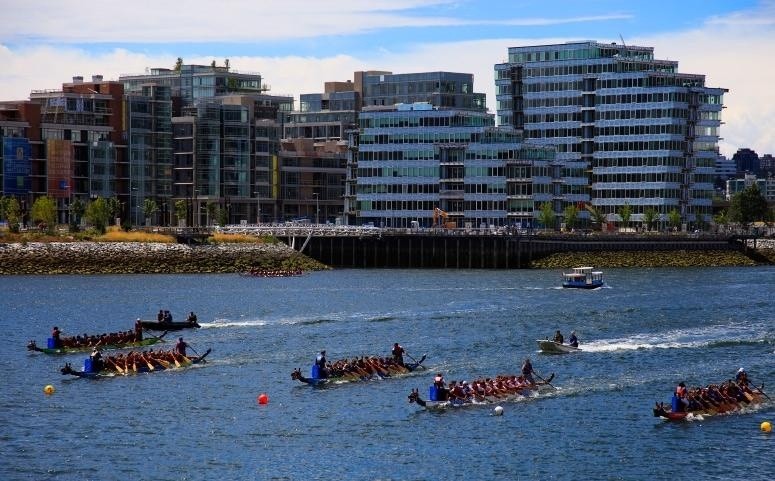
[187,311,196,323]
[157,310,164,322]
[553,330,563,344]
[316,342,405,378]
[677,367,753,412]
[164,310,172,321]
[52,317,188,370]
[568,329,578,347]
[248,266,302,276]
[433,358,536,401]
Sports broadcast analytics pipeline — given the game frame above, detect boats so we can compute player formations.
[408,373,554,410]
[237,270,311,278]
[537,340,581,353]
[61,354,203,379]
[143,319,201,330]
[28,330,167,355]
[291,354,426,387]
[653,382,765,420]
[563,266,603,288]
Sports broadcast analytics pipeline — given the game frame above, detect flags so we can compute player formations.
[434,207,448,219]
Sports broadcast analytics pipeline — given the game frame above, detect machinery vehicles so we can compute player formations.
[578,202,615,233]
[432,208,456,228]
